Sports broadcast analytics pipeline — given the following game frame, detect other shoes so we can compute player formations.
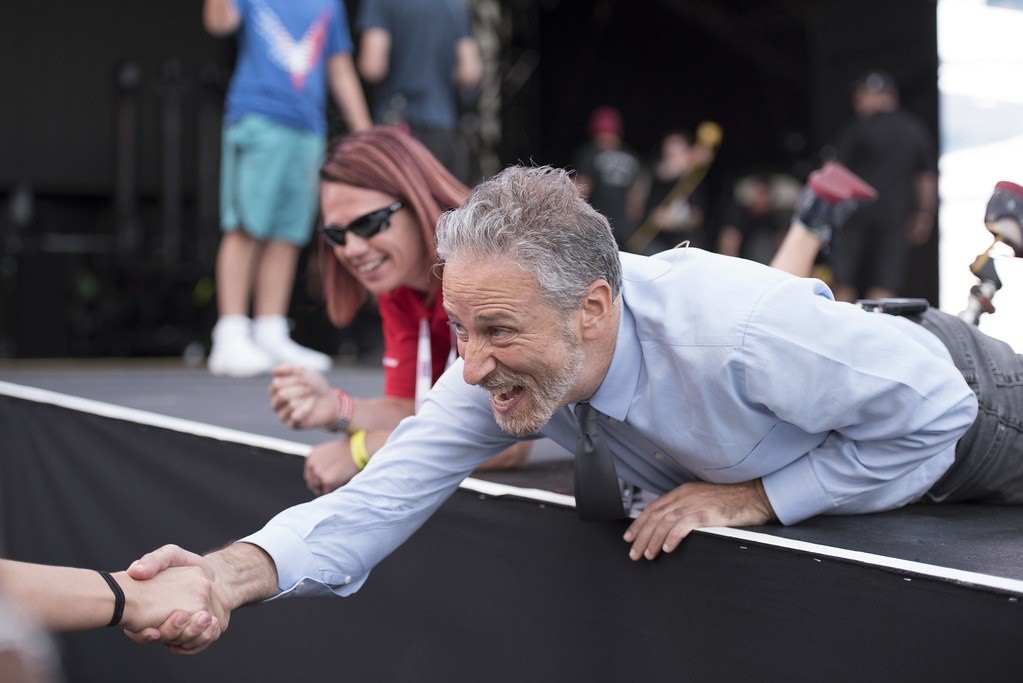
[984,181,1023,258]
[208,314,270,378]
[253,316,330,373]
[794,161,880,228]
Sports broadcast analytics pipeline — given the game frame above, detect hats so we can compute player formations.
[593,109,619,134]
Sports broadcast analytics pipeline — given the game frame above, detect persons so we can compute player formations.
[347,0,480,190]
[123,167,1023,656]
[201,1,372,378]
[575,70,936,300]
[269,128,1023,498]
[0,557,233,683]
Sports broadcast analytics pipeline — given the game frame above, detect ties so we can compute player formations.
[573,402,633,523]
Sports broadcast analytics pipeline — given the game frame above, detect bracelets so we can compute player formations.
[351,430,368,470]
[98,569,125,627]
[324,390,353,433]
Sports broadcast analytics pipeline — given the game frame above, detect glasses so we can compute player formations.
[317,199,406,246]
